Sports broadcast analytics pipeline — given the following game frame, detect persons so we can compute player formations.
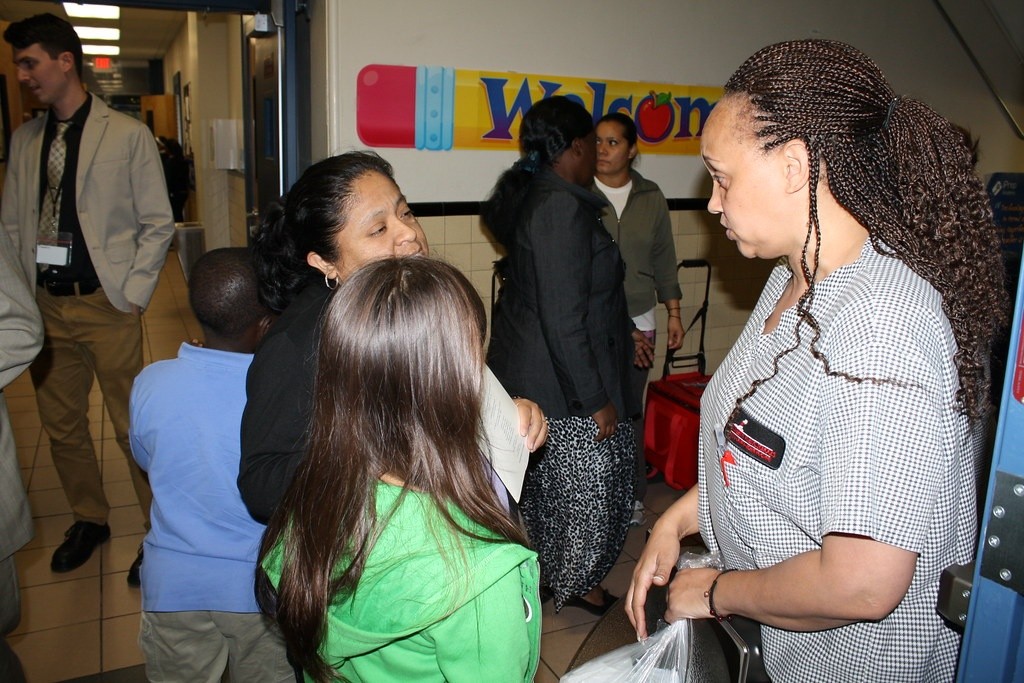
[0,97,686,683]
[0,16,175,587]
[625,39,1013,683]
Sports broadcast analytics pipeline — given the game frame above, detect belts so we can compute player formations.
[35,276,102,297]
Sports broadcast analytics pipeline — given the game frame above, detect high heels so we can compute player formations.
[537,585,555,604]
[561,579,619,616]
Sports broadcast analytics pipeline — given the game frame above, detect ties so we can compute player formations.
[36,122,73,272]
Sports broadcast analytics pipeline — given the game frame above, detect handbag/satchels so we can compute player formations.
[557,552,722,683]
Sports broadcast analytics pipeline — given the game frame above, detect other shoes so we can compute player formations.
[127,544,143,586]
[49,521,112,573]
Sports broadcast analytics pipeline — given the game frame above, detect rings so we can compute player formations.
[542,419,549,424]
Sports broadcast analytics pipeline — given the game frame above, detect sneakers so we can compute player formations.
[629,500,647,526]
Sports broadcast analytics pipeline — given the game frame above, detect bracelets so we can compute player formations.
[669,315,680,319]
[509,395,522,400]
[667,307,680,311]
[704,568,739,621]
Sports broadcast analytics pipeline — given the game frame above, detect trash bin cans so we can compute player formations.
[175,221,204,279]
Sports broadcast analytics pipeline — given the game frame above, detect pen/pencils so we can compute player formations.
[719,450,737,488]
